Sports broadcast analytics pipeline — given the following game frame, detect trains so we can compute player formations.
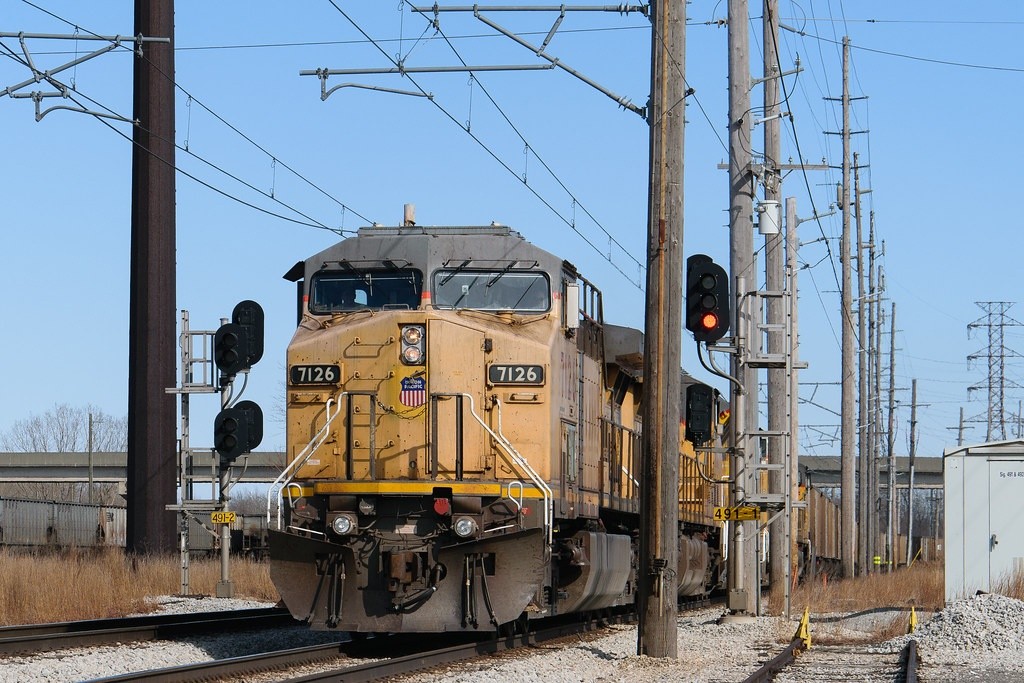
[275,218,943,636]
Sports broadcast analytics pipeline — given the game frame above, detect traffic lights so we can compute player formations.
[687,254,729,344]
[214,408,248,460]
[215,324,249,376]
